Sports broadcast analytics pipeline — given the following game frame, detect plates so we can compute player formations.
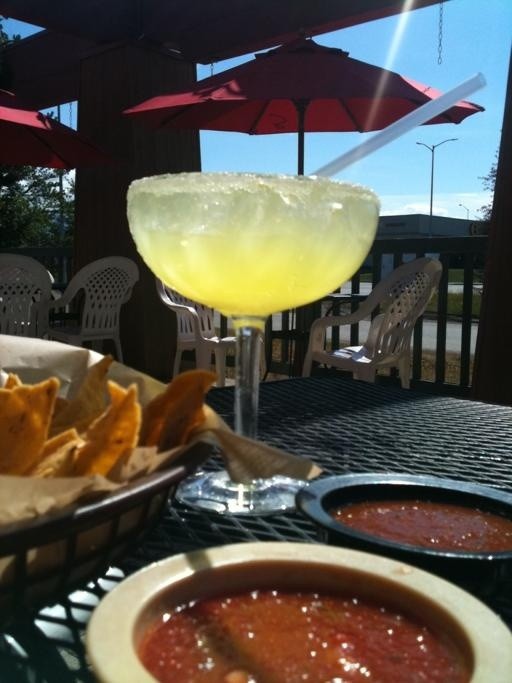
[0,333,214,559]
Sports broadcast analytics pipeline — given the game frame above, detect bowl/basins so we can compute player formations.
[86,541,512,683]
[295,473,512,631]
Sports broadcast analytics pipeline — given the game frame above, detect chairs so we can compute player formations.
[1,255,47,340]
[303,255,443,387]
[156,278,268,387]
[45,255,139,367]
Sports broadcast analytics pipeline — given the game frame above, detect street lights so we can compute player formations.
[459,203,470,220]
[416,138,459,236]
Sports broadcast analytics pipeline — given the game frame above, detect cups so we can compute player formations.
[128,172,380,518]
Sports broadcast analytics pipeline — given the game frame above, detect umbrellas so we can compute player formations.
[1,90,125,175]
[123,36,483,175]
